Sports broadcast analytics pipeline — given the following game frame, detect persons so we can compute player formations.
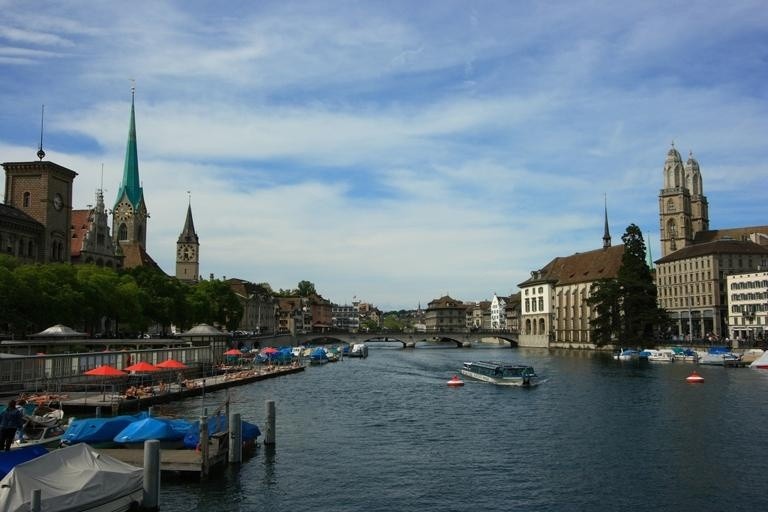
[223,370,259,381]
[0,391,69,452]
[268,361,274,369]
[177,378,196,390]
[118,381,165,399]
[220,361,229,368]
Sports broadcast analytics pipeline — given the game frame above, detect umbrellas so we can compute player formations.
[262,347,277,371]
[124,359,192,385]
[83,366,126,401]
[223,348,243,367]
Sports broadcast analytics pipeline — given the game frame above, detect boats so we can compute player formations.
[248,343,369,364]
[460,360,538,386]
[614,347,740,365]
[447,375,464,386]
[10,405,65,447]
[686,374,705,383]
